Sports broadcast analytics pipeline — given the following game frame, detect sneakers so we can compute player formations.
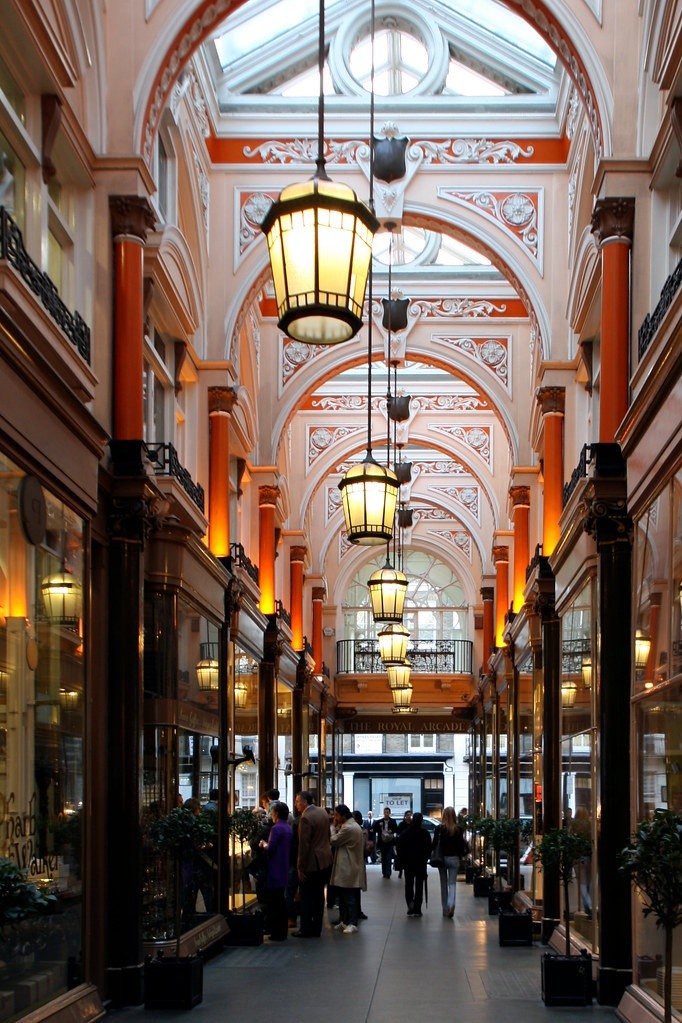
[334,922,347,929]
[344,924,359,934]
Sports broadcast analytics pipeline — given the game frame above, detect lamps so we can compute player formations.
[582,656,593,688]
[261,0,381,346]
[40,508,81,627]
[233,646,248,708]
[197,615,218,693]
[560,602,578,708]
[338,1,414,709]
[633,507,652,669]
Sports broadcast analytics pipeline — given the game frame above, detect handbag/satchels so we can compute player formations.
[429,824,443,867]
[381,831,393,843]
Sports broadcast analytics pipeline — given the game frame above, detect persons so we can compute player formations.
[560,808,574,884]
[568,807,594,920]
[396,811,412,879]
[364,810,378,864]
[182,798,213,917]
[430,806,463,917]
[199,789,219,912]
[248,786,368,941]
[404,813,432,917]
[173,792,185,814]
[376,808,397,879]
[458,808,470,838]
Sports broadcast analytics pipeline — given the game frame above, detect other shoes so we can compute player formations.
[443,911,448,916]
[448,909,454,918]
[414,910,423,916]
[407,910,414,916]
[360,912,367,919]
[331,920,340,925]
[292,929,320,938]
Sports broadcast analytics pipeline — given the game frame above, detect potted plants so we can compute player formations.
[138,808,212,1011]
[462,808,533,947]
[533,824,594,1006]
[230,804,273,946]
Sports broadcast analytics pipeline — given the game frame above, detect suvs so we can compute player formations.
[500,815,535,893]
[372,816,441,863]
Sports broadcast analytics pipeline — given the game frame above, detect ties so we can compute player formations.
[370,818,371,825]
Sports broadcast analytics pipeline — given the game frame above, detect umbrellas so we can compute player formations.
[424,862,428,908]
